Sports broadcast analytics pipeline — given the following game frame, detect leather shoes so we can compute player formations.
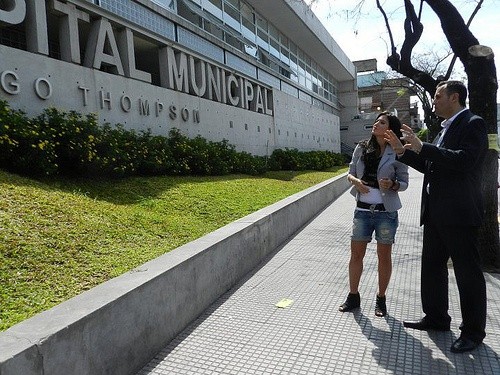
[403,317,450,332]
[451,336,483,352]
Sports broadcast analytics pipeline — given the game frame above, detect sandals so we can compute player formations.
[339,292,360,311]
[375,295,387,316]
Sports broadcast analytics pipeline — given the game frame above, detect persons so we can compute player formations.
[338,110,409,316]
[384,81,488,354]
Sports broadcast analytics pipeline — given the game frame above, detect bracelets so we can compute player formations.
[389,179,395,190]
[351,178,356,184]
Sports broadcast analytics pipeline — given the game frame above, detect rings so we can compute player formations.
[385,186,387,188]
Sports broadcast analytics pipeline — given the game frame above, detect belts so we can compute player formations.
[357,201,386,211]
